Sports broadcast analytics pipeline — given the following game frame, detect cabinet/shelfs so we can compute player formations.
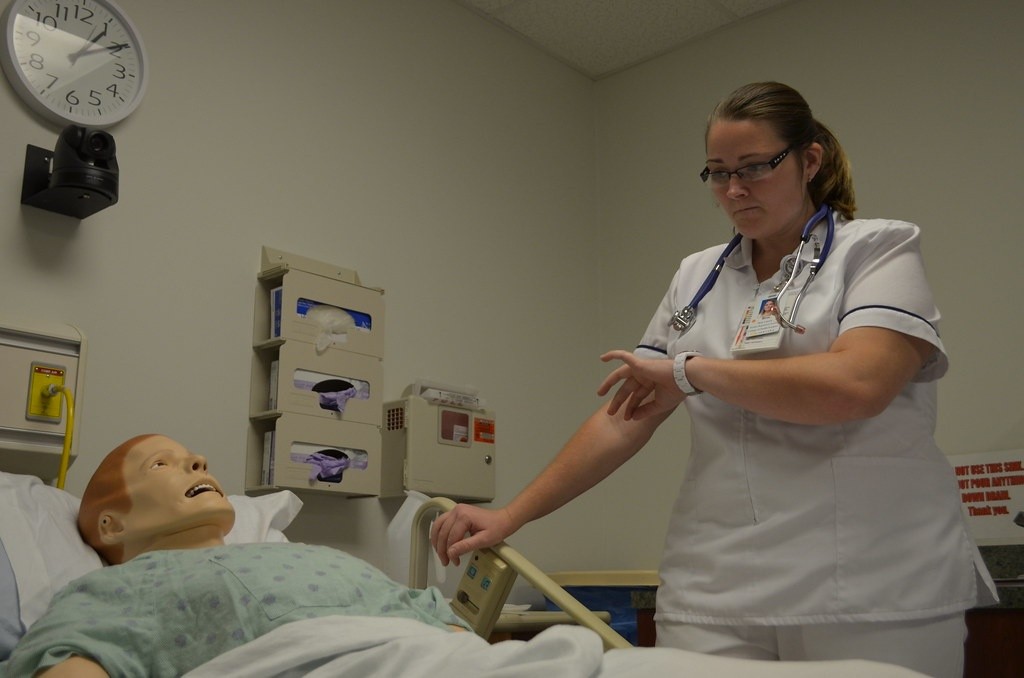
[244,245,385,499]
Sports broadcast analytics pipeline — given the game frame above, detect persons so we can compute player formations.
[758,299,775,320]
[430,82,1000,678]
[0,433,476,678]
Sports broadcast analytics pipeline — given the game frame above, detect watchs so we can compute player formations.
[673,350,704,396]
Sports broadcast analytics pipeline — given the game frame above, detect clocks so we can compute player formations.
[0,0,149,130]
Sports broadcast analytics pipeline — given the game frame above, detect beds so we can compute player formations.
[1,468,931,678]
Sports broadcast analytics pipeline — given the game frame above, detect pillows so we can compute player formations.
[0,470,303,660]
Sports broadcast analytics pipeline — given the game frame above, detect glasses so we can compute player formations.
[700,142,794,183]
